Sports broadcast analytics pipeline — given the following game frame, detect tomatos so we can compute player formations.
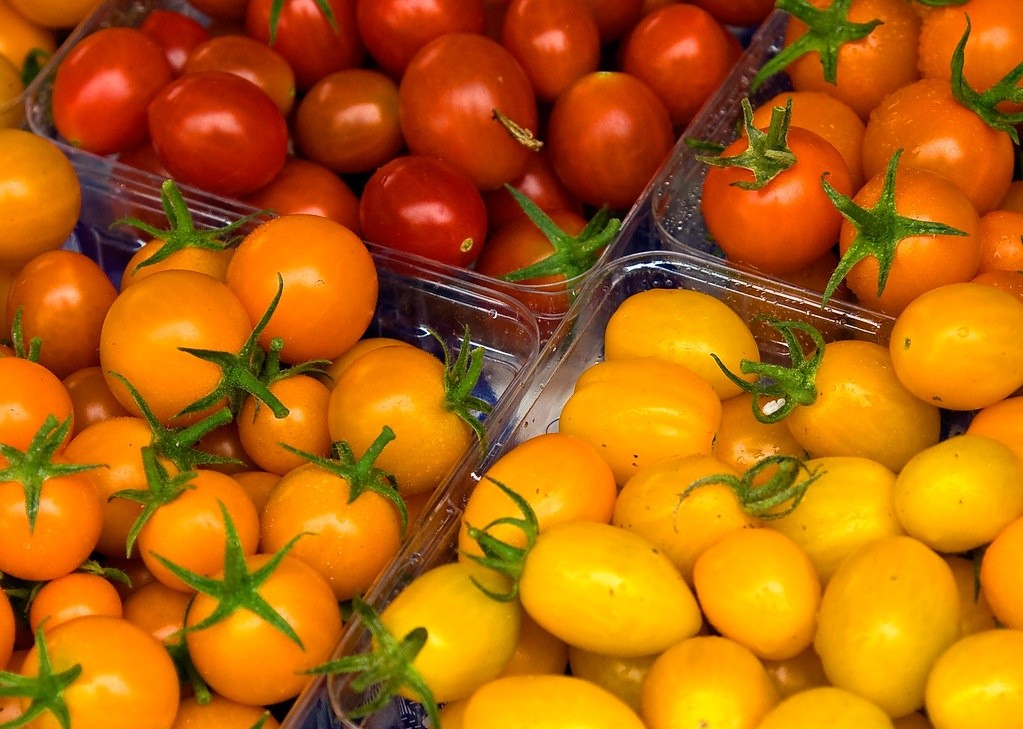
[2,0,1023,728]
